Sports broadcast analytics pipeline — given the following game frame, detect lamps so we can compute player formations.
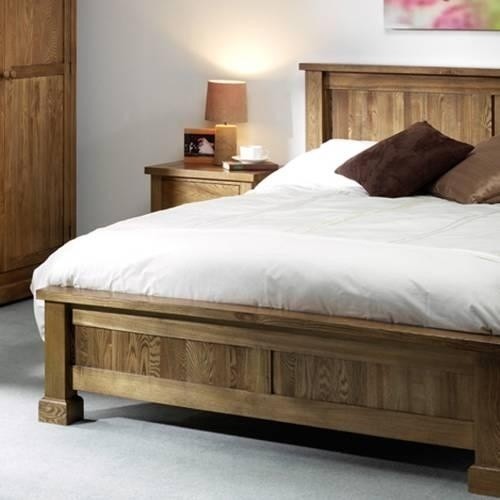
[204,78,246,165]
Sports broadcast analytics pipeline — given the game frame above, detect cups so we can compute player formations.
[240,145,264,158]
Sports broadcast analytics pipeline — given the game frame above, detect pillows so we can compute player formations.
[430,135,499,203]
[335,120,475,197]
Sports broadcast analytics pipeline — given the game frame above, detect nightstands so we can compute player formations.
[143,159,283,212]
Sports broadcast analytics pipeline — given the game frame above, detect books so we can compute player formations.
[222,158,279,172]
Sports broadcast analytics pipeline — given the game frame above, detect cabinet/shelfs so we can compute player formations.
[0,0,76,306]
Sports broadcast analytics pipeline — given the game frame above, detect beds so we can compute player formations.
[29,62,500,496]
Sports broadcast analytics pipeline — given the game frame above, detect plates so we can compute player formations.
[231,154,266,166]
